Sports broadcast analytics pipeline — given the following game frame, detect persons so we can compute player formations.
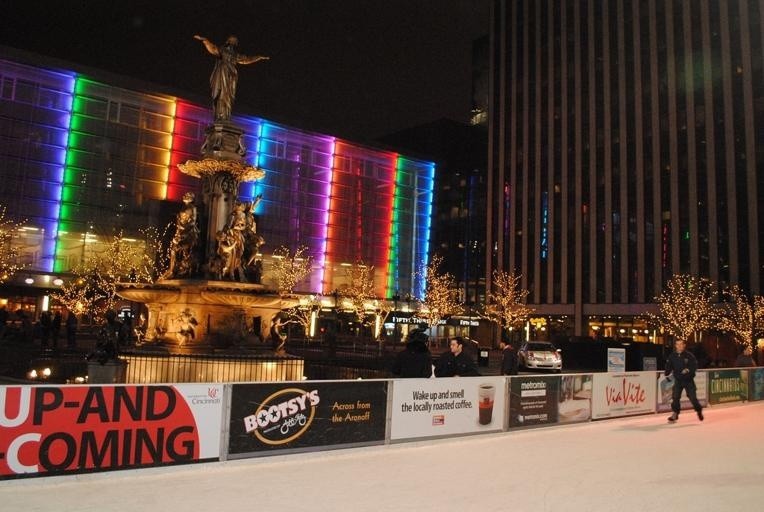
[156,189,197,276]
[0,302,77,346]
[432,336,472,377]
[193,33,269,123]
[214,227,237,276]
[391,333,433,377]
[499,338,517,375]
[222,197,246,282]
[664,337,703,421]
[244,194,265,256]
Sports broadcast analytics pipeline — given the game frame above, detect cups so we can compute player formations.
[479,383,495,425]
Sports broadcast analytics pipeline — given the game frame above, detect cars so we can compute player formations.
[516,340,563,370]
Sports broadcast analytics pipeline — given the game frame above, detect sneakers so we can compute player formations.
[698,412,704,421]
[668,412,678,420]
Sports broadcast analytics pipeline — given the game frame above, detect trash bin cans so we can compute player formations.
[478,349,489,368]
